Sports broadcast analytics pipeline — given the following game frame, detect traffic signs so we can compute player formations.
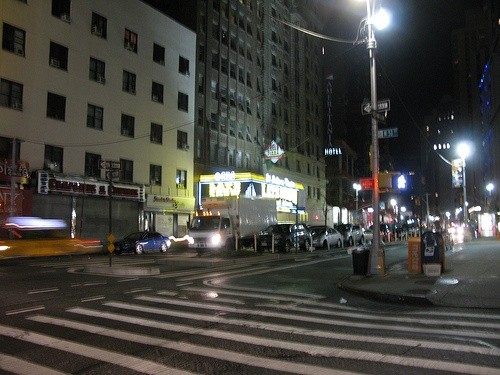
[98,158,121,179]
[361,99,390,124]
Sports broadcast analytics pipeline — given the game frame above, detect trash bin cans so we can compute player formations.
[407,237,421,272]
[352,249,370,275]
[421,230,444,274]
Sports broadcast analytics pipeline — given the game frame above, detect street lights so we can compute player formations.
[353,183,362,213]
[368,8,389,272]
[459,141,471,227]
[486,183,494,212]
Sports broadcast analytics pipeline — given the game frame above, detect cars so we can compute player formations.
[114,232,172,257]
[306,225,345,251]
[255,223,314,256]
[334,222,425,245]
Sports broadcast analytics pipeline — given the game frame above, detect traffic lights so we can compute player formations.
[395,175,408,191]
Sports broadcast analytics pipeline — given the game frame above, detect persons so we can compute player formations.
[434,221,442,234]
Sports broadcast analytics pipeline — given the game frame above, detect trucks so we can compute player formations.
[186,196,277,256]
